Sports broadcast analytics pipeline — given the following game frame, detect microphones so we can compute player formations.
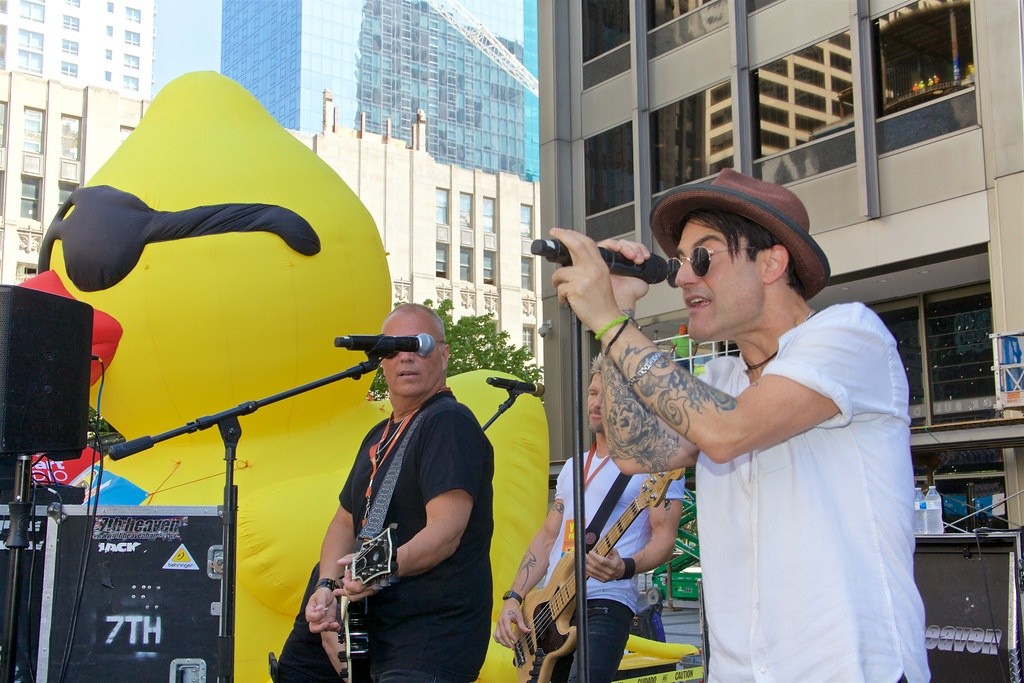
[486,377,545,397]
[531,237,669,284]
[334,333,435,357]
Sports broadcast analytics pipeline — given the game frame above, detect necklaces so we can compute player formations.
[378,412,410,454]
[746,310,819,370]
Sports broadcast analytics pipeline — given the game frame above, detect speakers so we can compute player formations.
[0,284,94,460]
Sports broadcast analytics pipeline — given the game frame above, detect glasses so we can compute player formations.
[663,247,751,286]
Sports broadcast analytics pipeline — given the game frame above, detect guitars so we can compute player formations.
[509,468,687,683]
[335,529,399,683]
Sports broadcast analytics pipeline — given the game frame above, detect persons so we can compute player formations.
[492,351,685,683]
[278,560,372,683]
[550,167,935,683]
[305,305,495,683]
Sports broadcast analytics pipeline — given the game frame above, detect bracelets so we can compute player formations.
[503,590,522,605]
[628,352,663,383]
[595,315,629,355]
[617,558,636,580]
[314,578,341,603]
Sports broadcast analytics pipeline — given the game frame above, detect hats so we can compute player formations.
[648,167,831,301]
